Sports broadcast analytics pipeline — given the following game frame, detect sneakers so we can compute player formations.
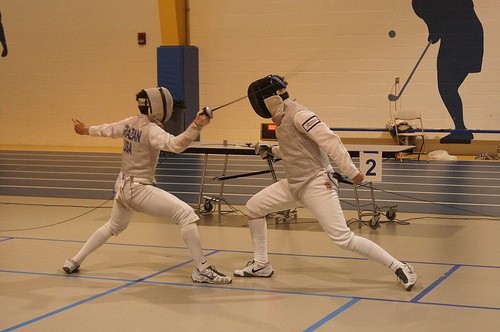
[191,264,232,283]
[233,260,276,277]
[62,258,80,274]
[397,261,418,291]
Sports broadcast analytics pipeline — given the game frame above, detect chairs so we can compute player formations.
[394,117,429,163]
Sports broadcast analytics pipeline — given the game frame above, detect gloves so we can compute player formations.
[194,110,210,128]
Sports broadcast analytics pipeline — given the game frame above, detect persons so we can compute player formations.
[233,75,417,291]
[63,86,233,284]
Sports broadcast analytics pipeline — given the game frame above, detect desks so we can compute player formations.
[183,142,417,230]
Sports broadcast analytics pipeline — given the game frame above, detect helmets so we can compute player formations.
[247,74,288,127]
[137,87,173,123]
